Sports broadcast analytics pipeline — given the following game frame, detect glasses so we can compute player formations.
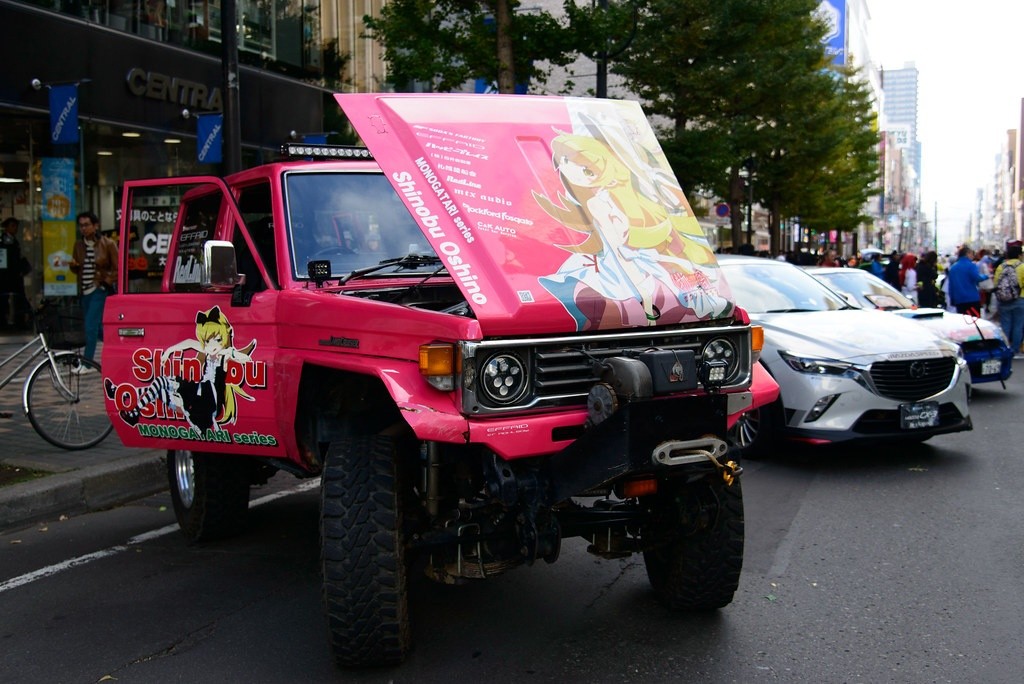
[79,223,93,228]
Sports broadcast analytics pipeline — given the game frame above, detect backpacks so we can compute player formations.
[996,261,1024,303]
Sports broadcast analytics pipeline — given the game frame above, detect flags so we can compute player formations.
[50,84,80,145]
[303,134,327,162]
[197,115,223,164]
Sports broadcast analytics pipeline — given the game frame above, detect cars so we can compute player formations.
[704,254,973,466]
[797,263,1013,383]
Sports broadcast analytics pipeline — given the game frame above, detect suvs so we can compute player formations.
[100,89,766,670]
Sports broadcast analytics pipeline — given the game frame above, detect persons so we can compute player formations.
[68,211,119,375]
[0,217,34,330]
[714,240,1024,361]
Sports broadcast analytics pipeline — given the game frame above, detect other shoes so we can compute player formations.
[71,365,97,375]
[1013,352,1024,360]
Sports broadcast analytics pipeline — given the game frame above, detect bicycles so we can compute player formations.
[0,289,114,450]
